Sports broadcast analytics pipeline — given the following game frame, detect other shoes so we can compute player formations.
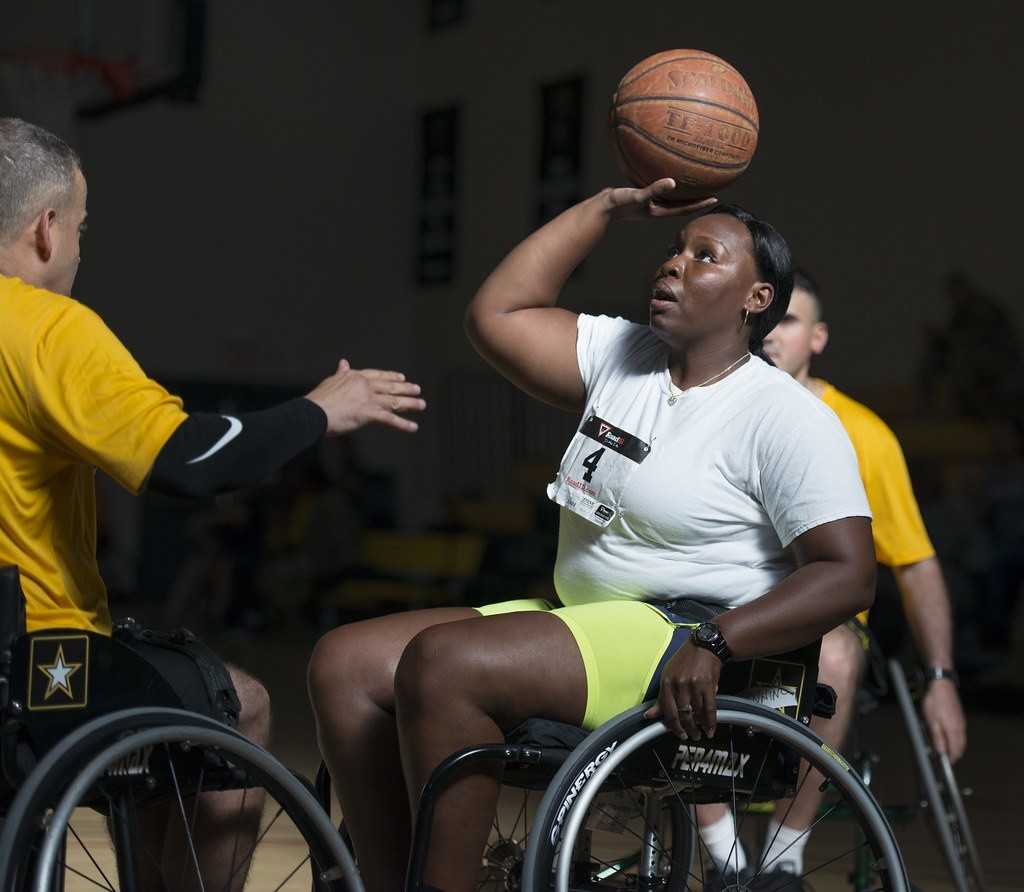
[706,869,805,892]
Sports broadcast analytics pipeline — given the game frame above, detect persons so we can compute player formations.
[0,119,427,892]
[305,169,877,892]
[685,269,966,892]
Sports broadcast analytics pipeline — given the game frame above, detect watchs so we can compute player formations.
[692,622,734,666]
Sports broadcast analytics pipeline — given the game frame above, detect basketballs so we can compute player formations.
[608,46,763,205]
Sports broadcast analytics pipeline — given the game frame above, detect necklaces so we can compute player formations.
[666,353,748,406]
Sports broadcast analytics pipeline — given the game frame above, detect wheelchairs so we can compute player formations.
[311,635,911,892]
[0,565,365,892]
[572,655,990,892]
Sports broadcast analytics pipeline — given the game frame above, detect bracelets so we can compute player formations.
[928,667,955,679]
